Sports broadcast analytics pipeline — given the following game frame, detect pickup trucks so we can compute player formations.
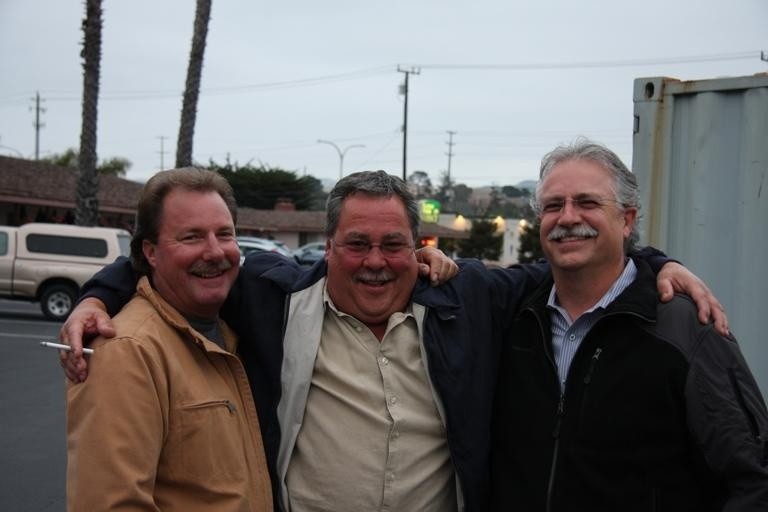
[0,223,131,322]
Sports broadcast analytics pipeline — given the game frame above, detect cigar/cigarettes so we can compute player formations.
[39,341,95,354]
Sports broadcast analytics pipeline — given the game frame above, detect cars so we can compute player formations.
[236,236,326,266]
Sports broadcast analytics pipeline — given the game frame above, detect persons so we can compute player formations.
[65,165,460,512]
[472,133,768,512]
[57,169,731,512]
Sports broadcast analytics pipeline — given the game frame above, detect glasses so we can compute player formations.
[543,196,620,211]
[333,235,413,256]
